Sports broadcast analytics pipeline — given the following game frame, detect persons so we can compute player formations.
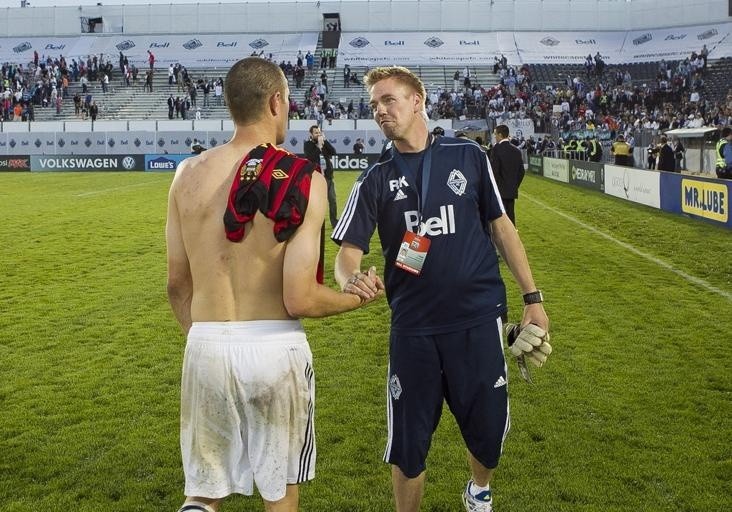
[486,125,525,228]
[329,64,551,512]
[166,58,381,511]
[304,125,338,228]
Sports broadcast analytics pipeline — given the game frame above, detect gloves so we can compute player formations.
[504,323,553,384]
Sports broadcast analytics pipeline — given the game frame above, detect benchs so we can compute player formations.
[286,67,370,107]
[406,66,499,93]
[33,68,233,121]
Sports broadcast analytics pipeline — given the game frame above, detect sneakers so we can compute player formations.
[462,478,494,511]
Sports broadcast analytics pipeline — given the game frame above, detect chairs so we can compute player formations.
[518,54,731,130]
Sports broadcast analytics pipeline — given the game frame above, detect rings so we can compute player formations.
[352,277,360,284]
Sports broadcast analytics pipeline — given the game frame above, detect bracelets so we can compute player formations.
[523,291,543,305]
